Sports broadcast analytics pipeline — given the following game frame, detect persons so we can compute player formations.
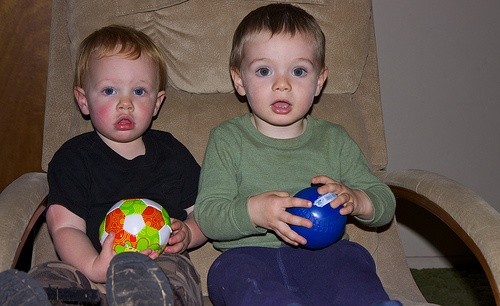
[0,24,209,306]
[193,3,404,306]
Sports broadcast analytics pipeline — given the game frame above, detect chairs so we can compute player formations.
[0,0,500,306]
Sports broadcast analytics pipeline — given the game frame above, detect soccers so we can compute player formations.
[99,198,171,256]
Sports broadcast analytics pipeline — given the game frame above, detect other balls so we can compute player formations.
[285,187,346,250]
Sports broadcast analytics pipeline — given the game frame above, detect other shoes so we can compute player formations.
[106,251,178,306]
[0,268,52,306]
